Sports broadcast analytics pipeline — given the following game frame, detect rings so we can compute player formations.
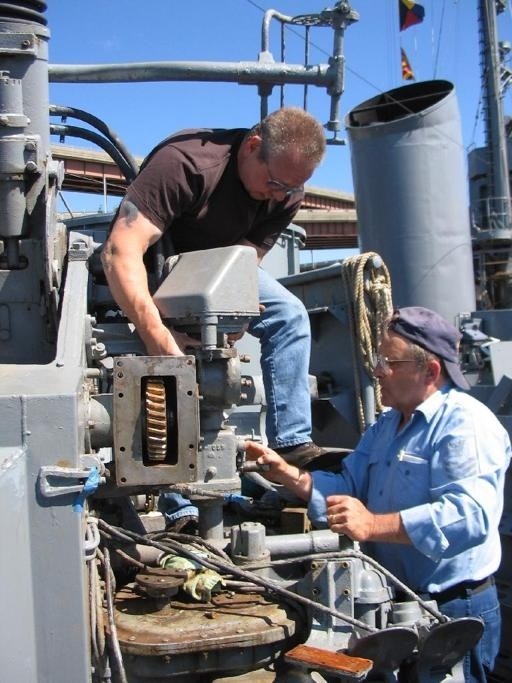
[331,513,337,524]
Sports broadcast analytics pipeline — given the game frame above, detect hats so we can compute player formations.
[387,306,471,391]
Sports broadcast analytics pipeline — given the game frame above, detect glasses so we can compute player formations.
[267,170,303,193]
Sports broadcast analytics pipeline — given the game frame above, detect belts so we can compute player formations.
[430,575,495,600]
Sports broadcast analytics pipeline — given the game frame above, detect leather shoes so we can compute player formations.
[278,443,342,471]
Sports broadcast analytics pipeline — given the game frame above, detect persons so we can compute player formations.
[241,306,511,682]
[100,106,353,473]
